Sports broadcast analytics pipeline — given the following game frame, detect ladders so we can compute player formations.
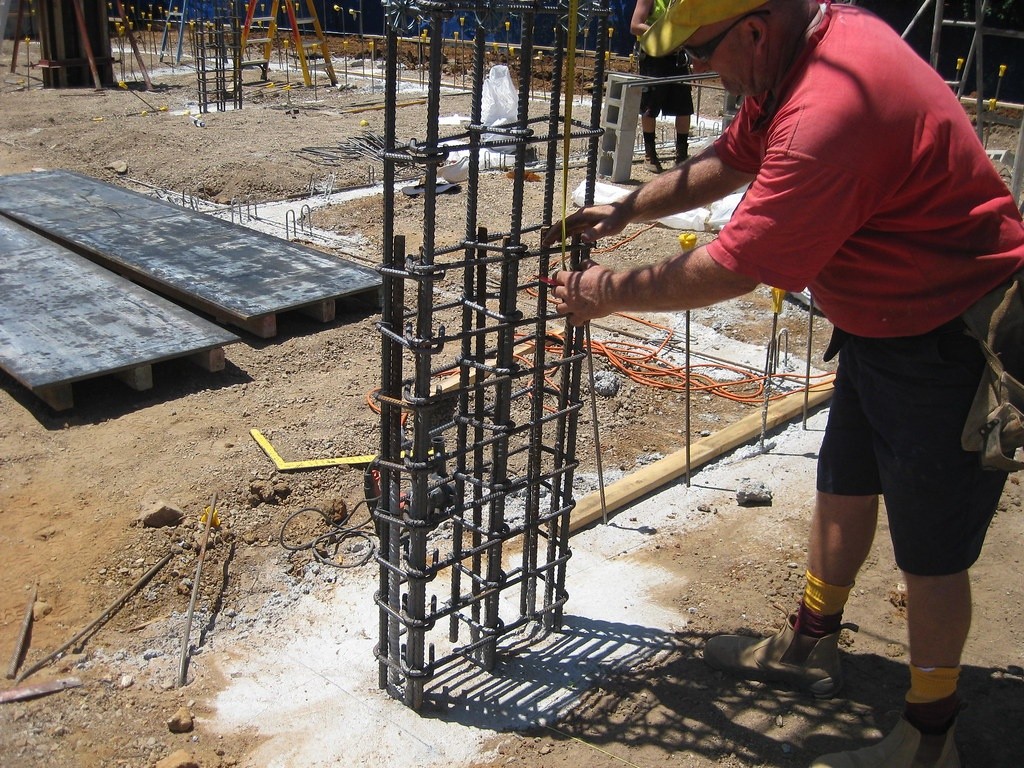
[234,0,338,89]
[932,0,1024,159]
[160,0,187,65]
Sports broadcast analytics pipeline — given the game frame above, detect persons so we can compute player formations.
[630,0,695,174]
[543,0,1024,768]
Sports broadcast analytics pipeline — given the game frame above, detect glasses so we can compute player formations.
[683,9,770,63]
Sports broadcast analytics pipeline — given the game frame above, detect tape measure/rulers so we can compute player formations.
[250,428,447,470]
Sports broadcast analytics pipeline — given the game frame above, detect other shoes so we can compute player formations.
[705,602,861,700]
[674,153,690,164]
[644,154,663,174]
[809,706,962,768]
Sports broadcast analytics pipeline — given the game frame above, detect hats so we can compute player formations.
[640,1,769,58]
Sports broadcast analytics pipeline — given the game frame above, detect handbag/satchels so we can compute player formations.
[961,277,1024,472]
